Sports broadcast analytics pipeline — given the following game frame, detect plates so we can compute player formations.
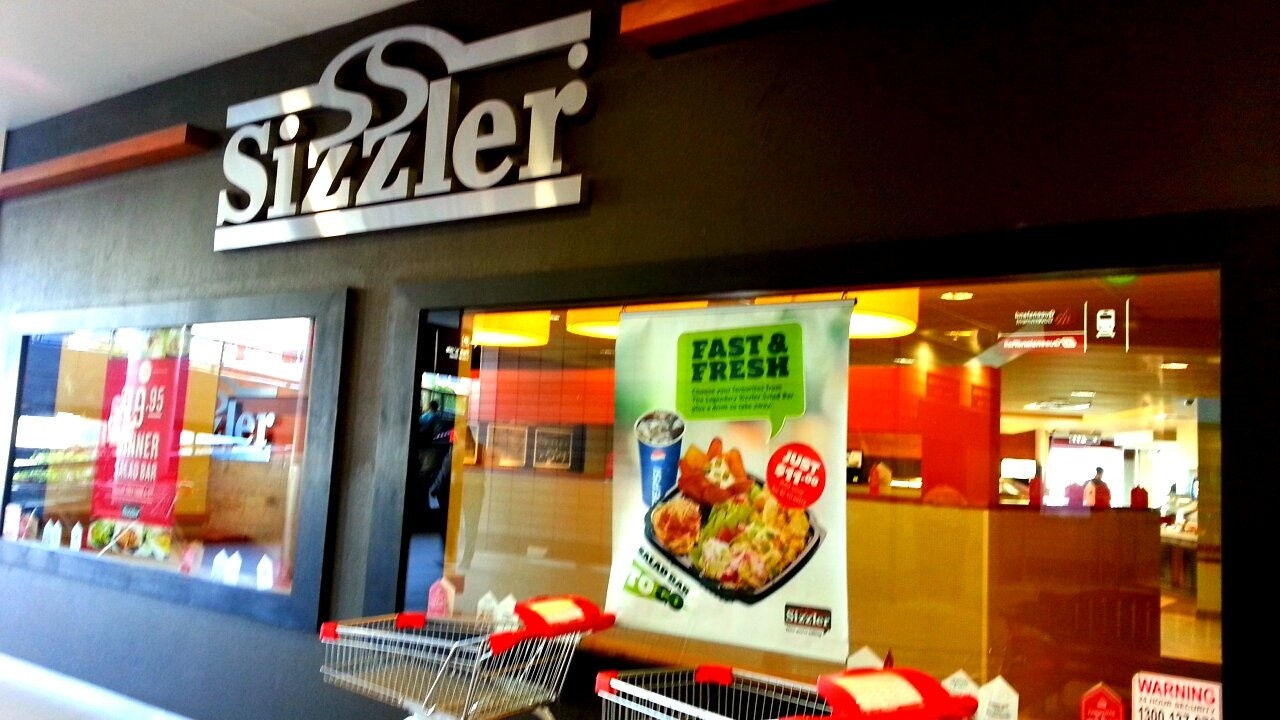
[645,467,828,607]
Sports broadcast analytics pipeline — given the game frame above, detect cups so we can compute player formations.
[634,408,685,507]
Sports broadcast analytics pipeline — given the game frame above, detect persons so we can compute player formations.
[854,445,1189,511]
[430,443,454,567]
[417,400,443,477]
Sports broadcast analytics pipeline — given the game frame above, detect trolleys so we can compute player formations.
[319,593,617,720]
[593,658,980,720]
